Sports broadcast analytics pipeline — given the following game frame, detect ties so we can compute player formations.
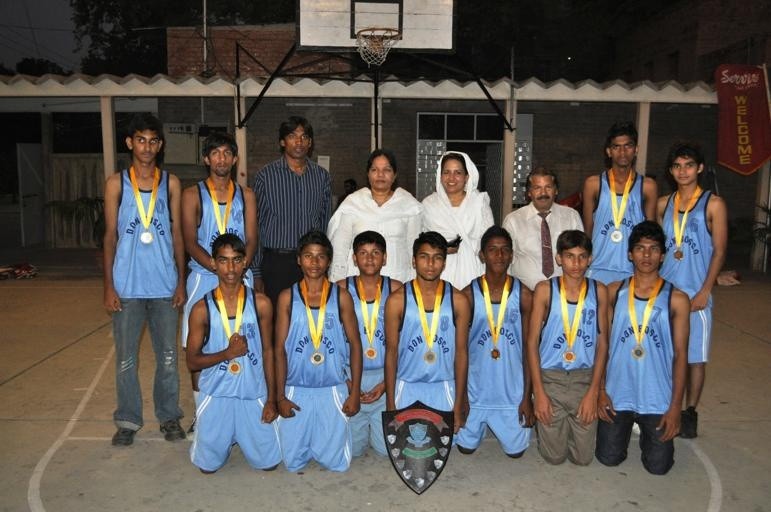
[536,213,555,279]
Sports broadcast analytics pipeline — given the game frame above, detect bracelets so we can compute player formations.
[266,398,278,402]
[278,395,286,405]
[601,386,605,390]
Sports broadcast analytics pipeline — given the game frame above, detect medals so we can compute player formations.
[565,351,576,362]
[230,359,242,377]
[141,232,153,244]
[425,351,436,363]
[491,349,501,360]
[366,349,377,359]
[633,345,645,358]
[312,352,324,365]
[675,250,683,260]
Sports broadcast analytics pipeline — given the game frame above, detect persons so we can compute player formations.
[183,116,659,324]
[274,228,365,474]
[333,232,402,458]
[185,232,284,473]
[526,229,609,464]
[596,221,691,475]
[458,229,537,459]
[102,111,186,445]
[653,141,731,441]
[383,231,473,446]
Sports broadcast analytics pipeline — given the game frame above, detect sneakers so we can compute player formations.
[110,426,136,445]
[677,410,704,441]
[159,419,186,442]
